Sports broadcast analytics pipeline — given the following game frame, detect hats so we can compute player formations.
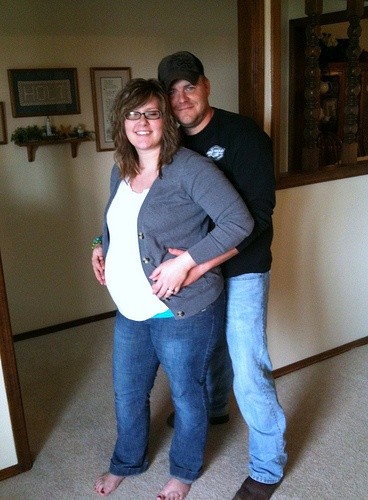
[158,51,204,93]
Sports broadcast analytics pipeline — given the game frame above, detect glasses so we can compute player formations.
[125,111,162,120]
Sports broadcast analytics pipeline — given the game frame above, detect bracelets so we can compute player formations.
[91,235,102,249]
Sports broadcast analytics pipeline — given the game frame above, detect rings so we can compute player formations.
[167,289,174,293]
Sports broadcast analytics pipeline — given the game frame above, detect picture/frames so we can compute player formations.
[7,68,81,118]
[89,67,132,152]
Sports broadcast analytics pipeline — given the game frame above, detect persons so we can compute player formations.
[152,51,288,500]
[90,79,255,500]
